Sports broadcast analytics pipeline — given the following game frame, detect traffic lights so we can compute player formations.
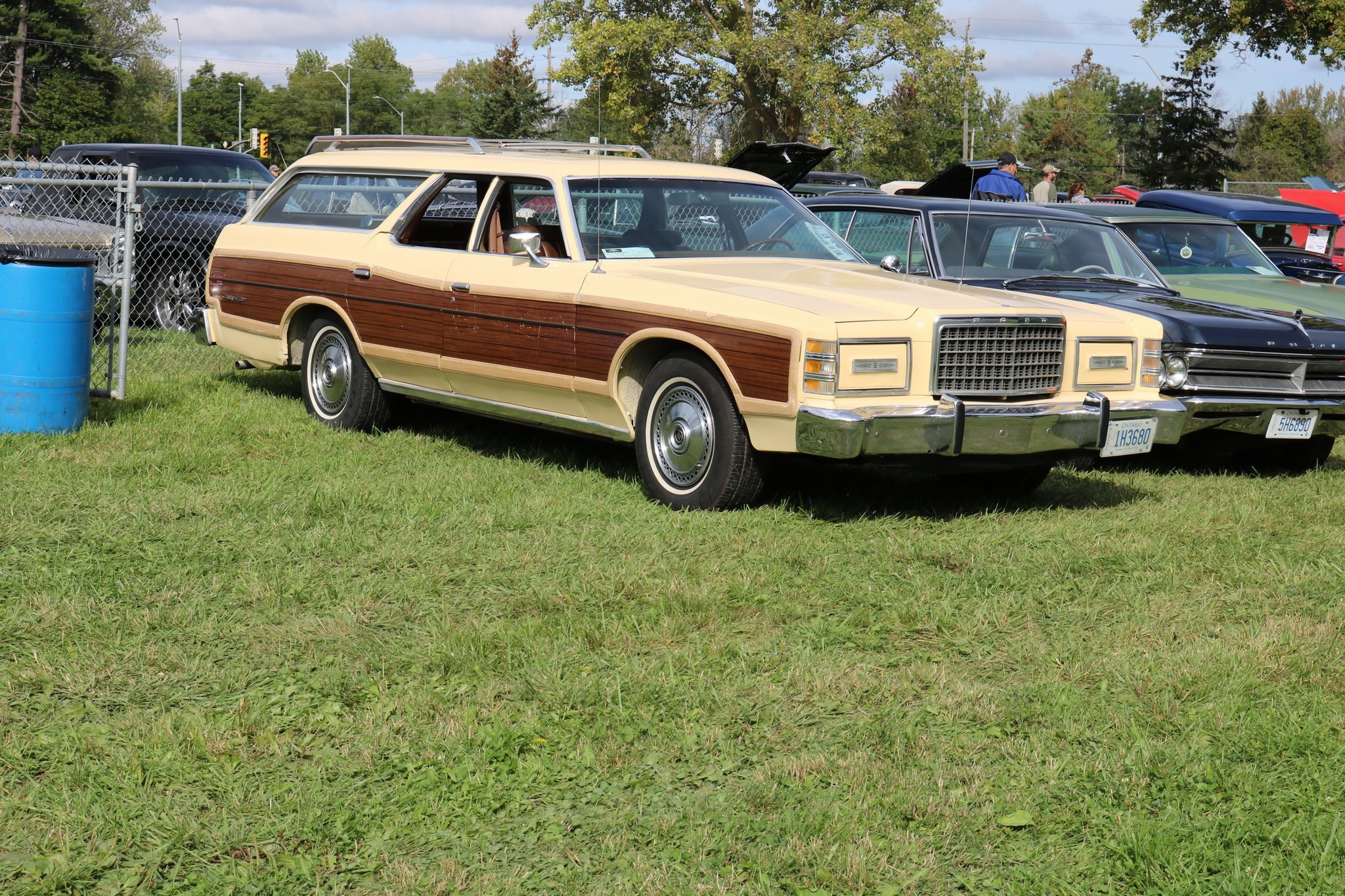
[260,133,268,158]
[250,128,259,149]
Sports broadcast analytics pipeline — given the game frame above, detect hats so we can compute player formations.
[998,152,1024,166]
[269,165,280,171]
[1042,164,1060,173]
[28,146,42,159]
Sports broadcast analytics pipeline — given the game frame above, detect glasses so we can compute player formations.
[1082,189,1086,192]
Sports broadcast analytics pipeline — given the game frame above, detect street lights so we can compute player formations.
[323,70,350,185]
[372,96,403,134]
[173,17,183,146]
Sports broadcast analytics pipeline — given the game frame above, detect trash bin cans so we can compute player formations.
[0,244,98,438]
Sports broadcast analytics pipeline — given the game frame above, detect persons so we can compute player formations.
[1067,183,1091,203]
[1030,164,1061,248]
[269,165,281,178]
[969,152,1027,247]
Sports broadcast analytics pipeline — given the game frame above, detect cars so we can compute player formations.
[720,175,1345,469]
[0,144,555,334]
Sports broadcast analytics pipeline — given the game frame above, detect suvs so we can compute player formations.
[195,138,1187,513]
